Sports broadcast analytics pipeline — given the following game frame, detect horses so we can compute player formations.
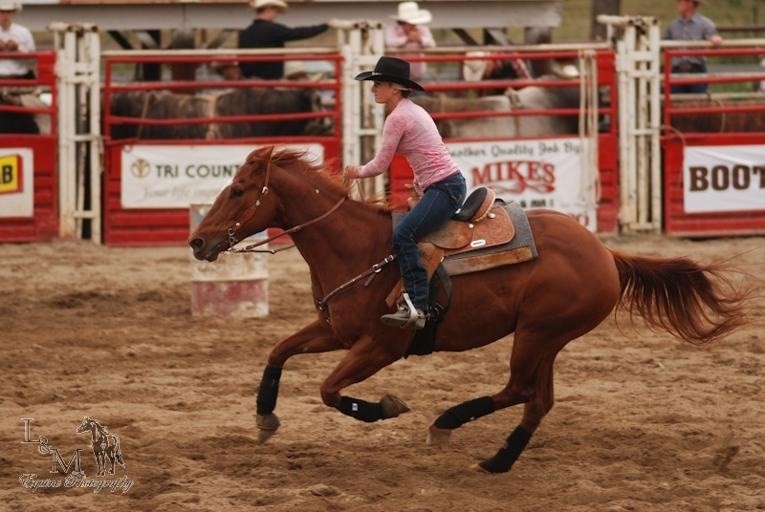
[188,144,765,476]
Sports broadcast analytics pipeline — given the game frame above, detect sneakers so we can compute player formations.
[380,307,426,330]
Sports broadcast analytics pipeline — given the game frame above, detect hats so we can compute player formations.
[0,0,22,14]
[354,56,425,92]
[388,2,433,25]
[249,0,288,13]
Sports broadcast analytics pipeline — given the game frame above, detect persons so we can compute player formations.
[344,56,466,331]
[0,0,51,133]
[662,0,725,93]
[236,0,534,98]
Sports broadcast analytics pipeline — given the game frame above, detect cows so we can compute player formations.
[384,64,606,139]
[87,70,335,139]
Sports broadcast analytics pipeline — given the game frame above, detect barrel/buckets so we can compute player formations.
[189,203,269,320]
